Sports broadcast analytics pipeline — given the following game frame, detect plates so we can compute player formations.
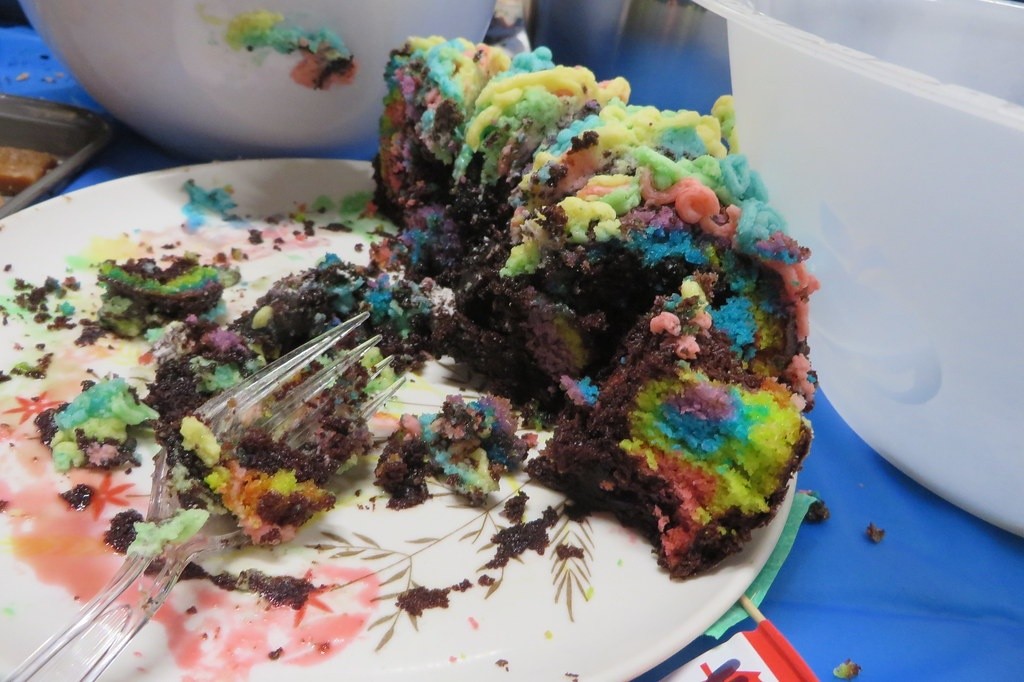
[0,160,797,682]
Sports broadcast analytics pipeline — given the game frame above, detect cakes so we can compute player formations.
[27,11,821,580]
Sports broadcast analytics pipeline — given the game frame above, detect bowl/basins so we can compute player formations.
[20,0,496,154]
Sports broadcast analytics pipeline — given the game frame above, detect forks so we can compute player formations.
[0,311,407,682]
